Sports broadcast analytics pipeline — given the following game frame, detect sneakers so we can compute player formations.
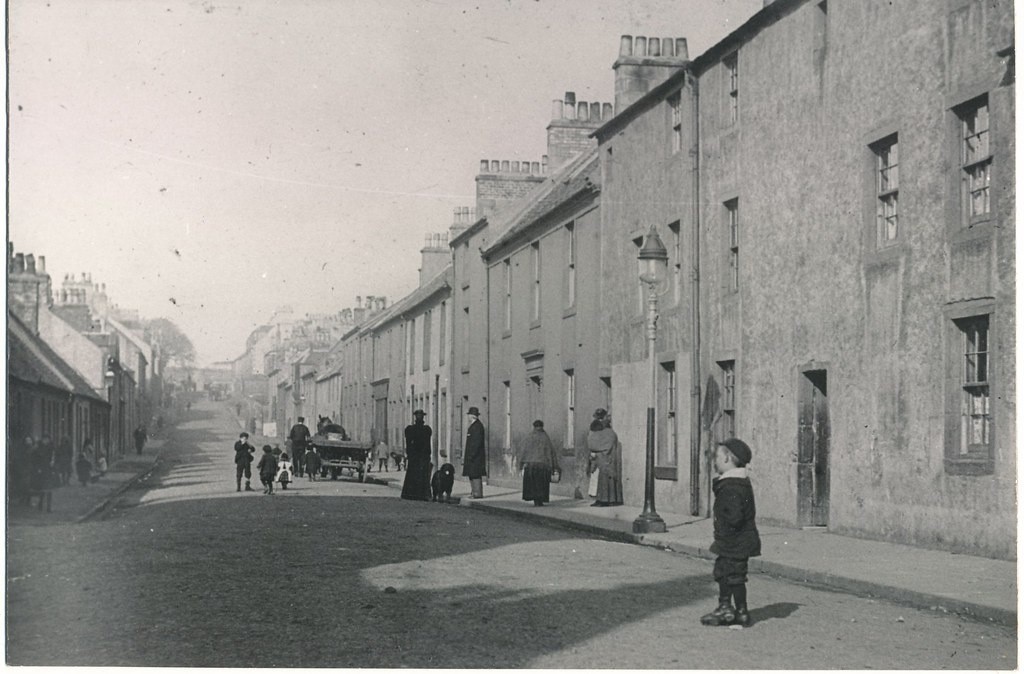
[700,604,750,626]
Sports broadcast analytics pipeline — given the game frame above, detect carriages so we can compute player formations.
[310,415,371,484]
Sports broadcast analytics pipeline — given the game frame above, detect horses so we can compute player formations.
[317,414,347,480]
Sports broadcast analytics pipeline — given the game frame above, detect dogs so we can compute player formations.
[431,462,455,502]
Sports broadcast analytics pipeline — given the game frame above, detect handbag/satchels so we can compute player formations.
[551,468,562,483]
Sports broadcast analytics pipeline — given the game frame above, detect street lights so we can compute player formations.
[634,222,669,534]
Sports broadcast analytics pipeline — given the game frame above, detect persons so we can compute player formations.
[258,416,321,494]
[234,433,255,492]
[401,409,433,500]
[587,408,623,506]
[133,424,148,455]
[375,439,406,473]
[516,419,558,508]
[462,406,487,499]
[700,438,761,627]
[14,435,108,513]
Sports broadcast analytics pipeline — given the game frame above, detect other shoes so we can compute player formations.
[267,491,275,495]
[245,486,255,491]
[300,473,303,477]
[590,500,609,507]
[263,488,269,494]
[236,487,241,492]
[295,472,298,477]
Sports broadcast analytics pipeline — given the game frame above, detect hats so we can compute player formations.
[412,410,426,415]
[720,438,753,463]
[466,407,481,416]
[297,417,304,422]
[592,408,609,419]
